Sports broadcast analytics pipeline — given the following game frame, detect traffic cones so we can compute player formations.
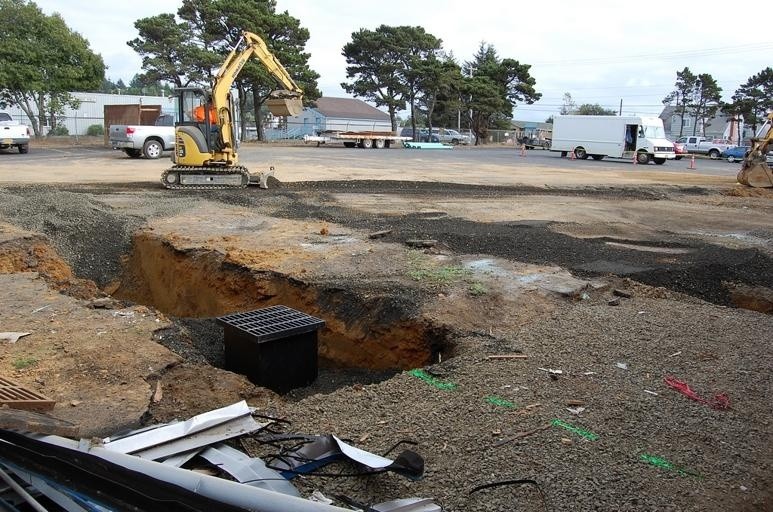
[568,147,575,161]
[518,143,526,157]
[630,151,637,167]
[686,153,696,170]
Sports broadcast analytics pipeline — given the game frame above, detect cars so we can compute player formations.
[516,133,550,149]
[667,135,772,165]
[399,126,476,145]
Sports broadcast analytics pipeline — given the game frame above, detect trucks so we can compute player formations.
[550,113,675,166]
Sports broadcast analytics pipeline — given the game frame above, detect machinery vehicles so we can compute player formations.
[734,112,772,188]
[160,29,305,189]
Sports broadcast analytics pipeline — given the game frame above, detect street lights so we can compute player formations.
[466,66,477,131]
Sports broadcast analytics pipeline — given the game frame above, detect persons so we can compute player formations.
[185,95,225,153]
[161,95,176,114]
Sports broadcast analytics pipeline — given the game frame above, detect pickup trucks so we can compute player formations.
[0,113,31,153]
[108,112,188,160]
